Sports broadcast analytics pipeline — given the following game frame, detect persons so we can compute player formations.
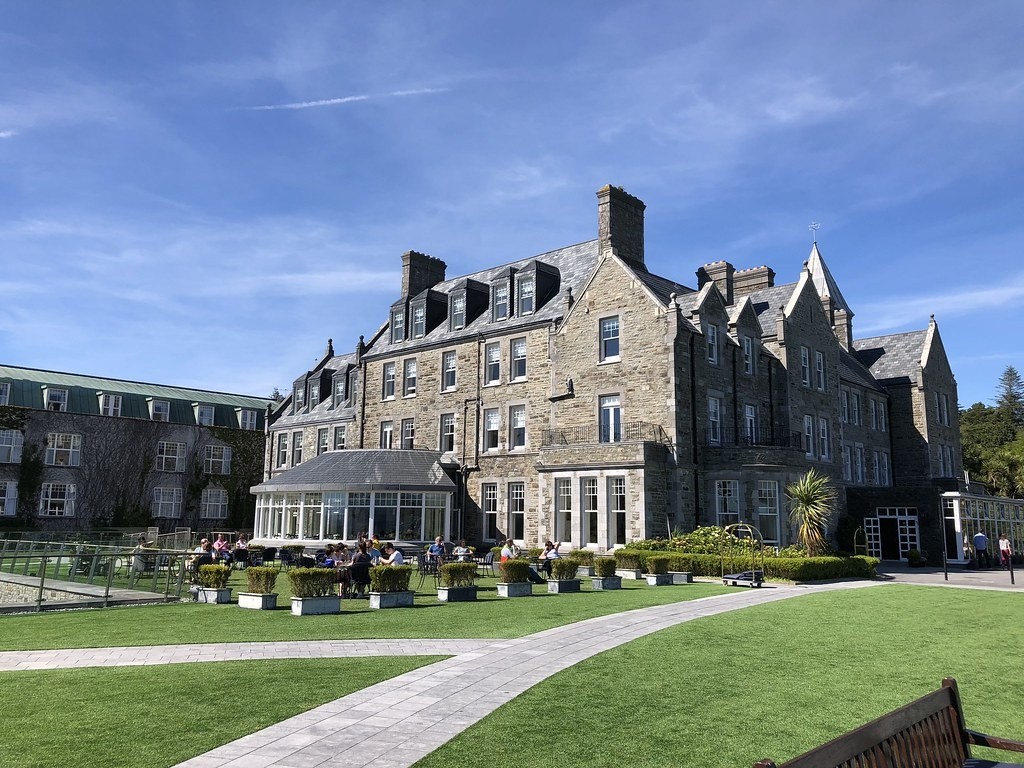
[129,536,154,572]
[452,539,473,575]
[321,533,403,599]
[214,533,236,569]
[427,536,444,575]
[191,538,215,585]
[500,538,547,584]
[999,534,1012,571]
[537,541,560,579]
[973,528,989,570]
[236,533,247,567]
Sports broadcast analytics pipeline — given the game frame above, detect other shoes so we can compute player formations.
[329,590,365,599]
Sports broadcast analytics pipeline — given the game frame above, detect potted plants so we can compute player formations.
[546,559,579,594]
[285,569,348,615]
[492,546,515,577]
[589,559,622,590]
[194,561,231,605]
[495,559,531,597]
[435,561,480,601]
[368,564,416,609]
[567,549,595,576]
[907,549,920,567]
[614,549,640,579]
[237,566,278,609]
[527,547,549,573]
[646,556,674,586]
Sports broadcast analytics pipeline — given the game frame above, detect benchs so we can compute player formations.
[751,676,1024,768]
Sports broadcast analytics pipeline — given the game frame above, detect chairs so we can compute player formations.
[71,549,562,601]
